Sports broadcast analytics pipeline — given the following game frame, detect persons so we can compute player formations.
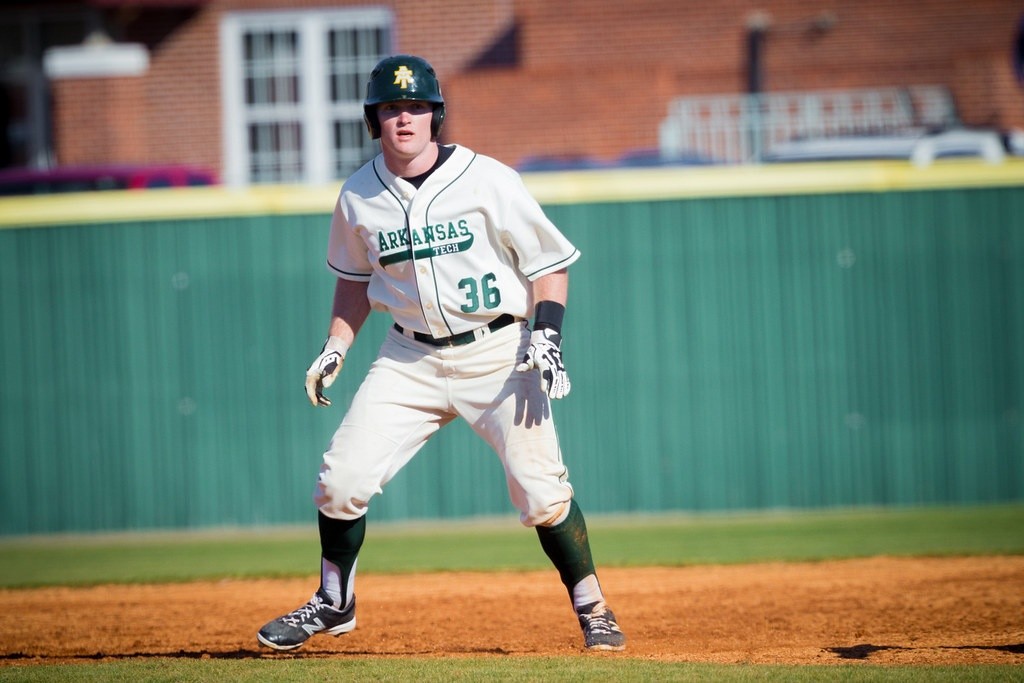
[257,56,635,652]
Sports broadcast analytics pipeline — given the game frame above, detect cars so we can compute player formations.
[519,153,705,173]
[746,127,1024,163]
[0,166,223,198]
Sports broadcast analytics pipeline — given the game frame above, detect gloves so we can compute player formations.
[305,336,351,408]
[516,324,571,400]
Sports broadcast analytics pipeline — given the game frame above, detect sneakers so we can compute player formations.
[578,604,626,651]
[257,586,357,650]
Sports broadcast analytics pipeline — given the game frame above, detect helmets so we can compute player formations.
[364,55,445,140]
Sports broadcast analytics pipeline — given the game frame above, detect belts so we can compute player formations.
[394,313,517,347]
